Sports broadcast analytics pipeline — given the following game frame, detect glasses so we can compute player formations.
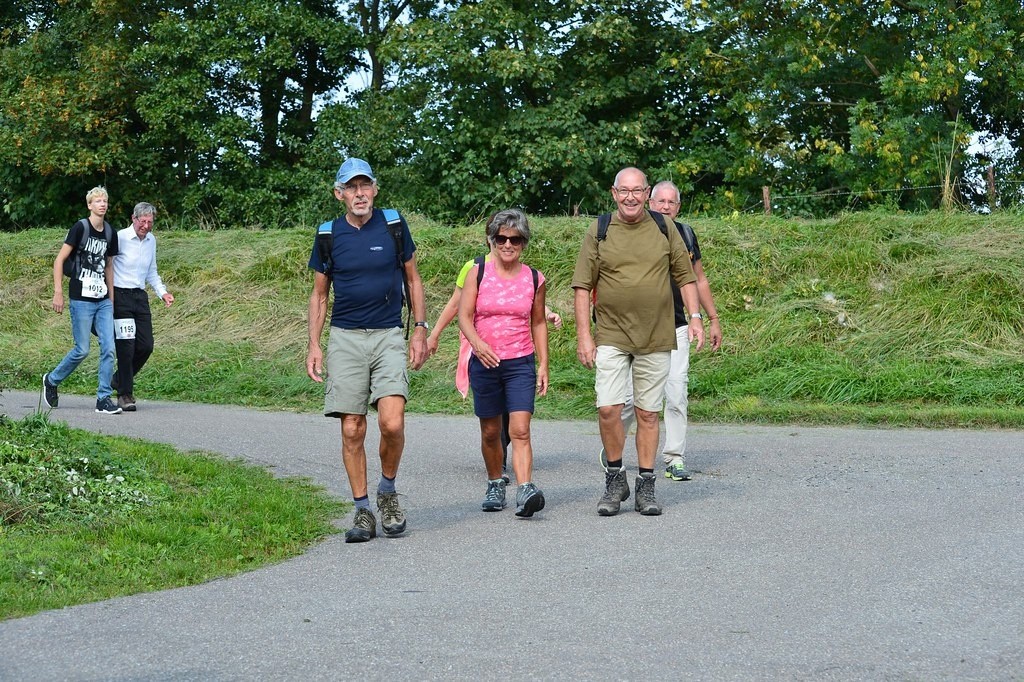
[493,235,523,246]
[136,217,153,226]
[613,186,649,198]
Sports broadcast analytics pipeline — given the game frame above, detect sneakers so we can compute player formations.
[481,479,507,511]
[42,373,59,409]
[117,394,136,411]
[514,483,545,518]
[377,491,407,537]
[111,376,118,390]
[600,447,608,469]
[345,506,376,542]
[95,396,123,414]
[665,463,691,481]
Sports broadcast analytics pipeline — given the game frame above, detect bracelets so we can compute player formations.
[709,314,719,320]
[690,312,702,320]
[546,311,552,320]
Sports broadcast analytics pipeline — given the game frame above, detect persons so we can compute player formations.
[114,202,174,412]
[427,210,561,487]
[571,168,705,515]
[42,184,122,414]
[600,180,723,480]
[458,210,549,518]
[306,157,426,543]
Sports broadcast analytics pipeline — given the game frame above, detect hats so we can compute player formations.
[336,157,376,183]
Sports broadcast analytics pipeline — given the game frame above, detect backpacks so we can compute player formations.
[63,218,111,280]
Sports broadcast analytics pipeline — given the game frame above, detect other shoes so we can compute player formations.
[502,472,510,484]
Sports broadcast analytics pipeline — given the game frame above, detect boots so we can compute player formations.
[597,466,630,515]
[635,472,661,515]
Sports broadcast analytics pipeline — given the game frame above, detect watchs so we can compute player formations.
[415,321,429,329]
